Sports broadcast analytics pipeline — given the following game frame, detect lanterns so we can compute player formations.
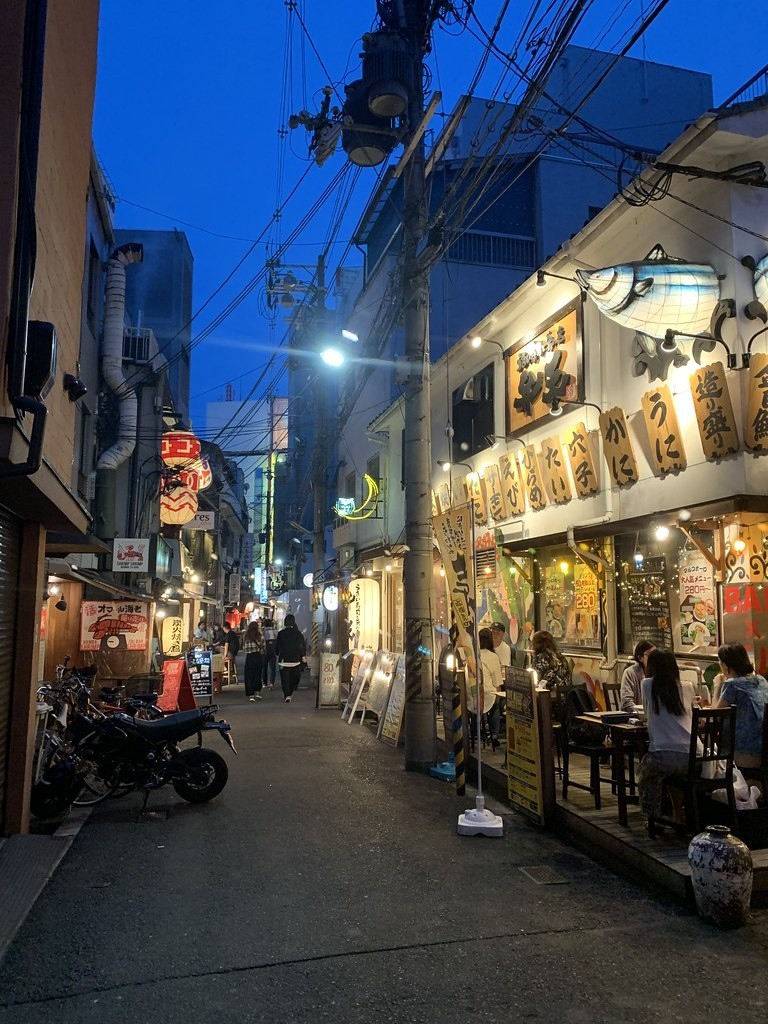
[185,459,214,492]
[225,611,241,630]
[348,578,381,656]
[162,616,183,657]
[159,465,200,525]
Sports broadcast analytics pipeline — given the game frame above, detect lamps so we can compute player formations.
[62,372,79,389]
[43,588,67,611]
[661,327,737,369]
[548,395,603,417]
[69,377,87,402]
[484,434,527,449]
[535,267,588,301]
[466,334,506,362]
[436,460,473,477]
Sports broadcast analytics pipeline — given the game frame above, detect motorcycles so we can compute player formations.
[29,665,239,818]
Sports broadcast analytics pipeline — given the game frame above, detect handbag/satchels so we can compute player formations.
[707,745,750,803]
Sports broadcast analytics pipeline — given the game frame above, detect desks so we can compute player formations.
[578,710,676,826]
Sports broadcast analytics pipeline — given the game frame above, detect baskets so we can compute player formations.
[126,672,164,698]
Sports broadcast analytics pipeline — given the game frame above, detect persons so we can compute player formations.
[464,627,504,750]
[620,638,659,763]
[194,621,208,640]
[711,639,768,769]
[212,621,239,694]
[261,619,278,691]
[529,630,574,722]
[158,429,202,469]
[488,621,511,715]
[636,648,705,836]
[275,614,307,703]
[242,621,267,702]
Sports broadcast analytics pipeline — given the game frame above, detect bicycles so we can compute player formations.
[37,656,203,806]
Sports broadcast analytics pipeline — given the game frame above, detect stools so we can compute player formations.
[470,705,498,753]
[223,659,238,688]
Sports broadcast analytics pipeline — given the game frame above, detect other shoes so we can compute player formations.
[262,684,266,688]
[492,734,500,747]
[255,693,262,699]
[249,696,256,702]
[269,684,273,687]
[644,818,665,833]
[285,696,291,703]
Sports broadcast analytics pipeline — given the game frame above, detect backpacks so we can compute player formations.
[564,689,605,745]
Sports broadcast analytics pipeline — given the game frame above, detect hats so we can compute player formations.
[489,622,505,632]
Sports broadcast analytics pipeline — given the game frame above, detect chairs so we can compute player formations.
[554,683,617,811]
[602,683,644,796]
[733,702,768,799]
[647,705,740,842]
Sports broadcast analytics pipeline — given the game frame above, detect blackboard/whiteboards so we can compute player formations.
[320,653,341,706]
[184,650,213,697]
[366,648,400,717]
[156,661,197,716]
[345,649,373,709]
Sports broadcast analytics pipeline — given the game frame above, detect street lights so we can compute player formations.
[316,346,437,771]
